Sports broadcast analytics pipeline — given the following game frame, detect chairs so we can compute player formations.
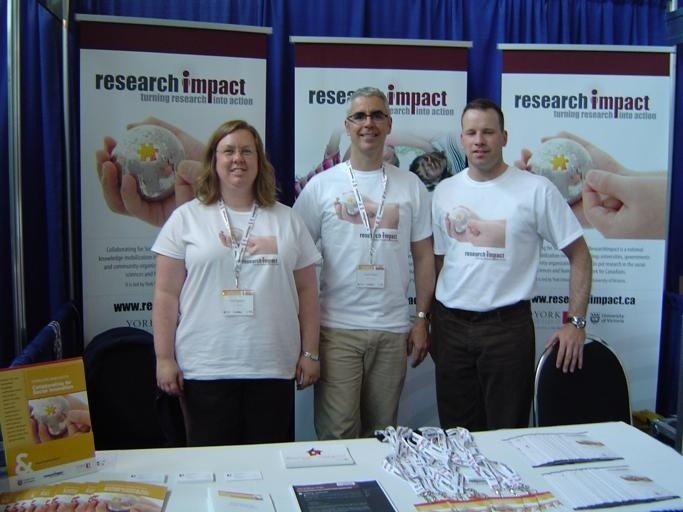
[83,327,188,449]
[534,332,634,427]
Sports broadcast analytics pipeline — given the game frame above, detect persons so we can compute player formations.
[151,120,322,447]
[295,122,470,199]
[97,116,211,228]
[432,98,591,432]
[445,205,506,249]
[30,392,93,443]
[219,227,277,255]
[333,189,399,229]
[516,133,667,240]
[291,87,437,439]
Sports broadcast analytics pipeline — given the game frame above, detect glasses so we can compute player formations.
[346,109,390,127]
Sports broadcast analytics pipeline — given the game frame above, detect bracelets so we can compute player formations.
[302,351,320,360]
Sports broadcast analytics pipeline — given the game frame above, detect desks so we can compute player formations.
[0,420,683,512]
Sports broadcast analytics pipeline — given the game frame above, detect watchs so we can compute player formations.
[417,312,432,320]
[566,314,586,327]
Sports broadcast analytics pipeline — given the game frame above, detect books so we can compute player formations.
[0,481,167,512]
[506,434,679,510]
